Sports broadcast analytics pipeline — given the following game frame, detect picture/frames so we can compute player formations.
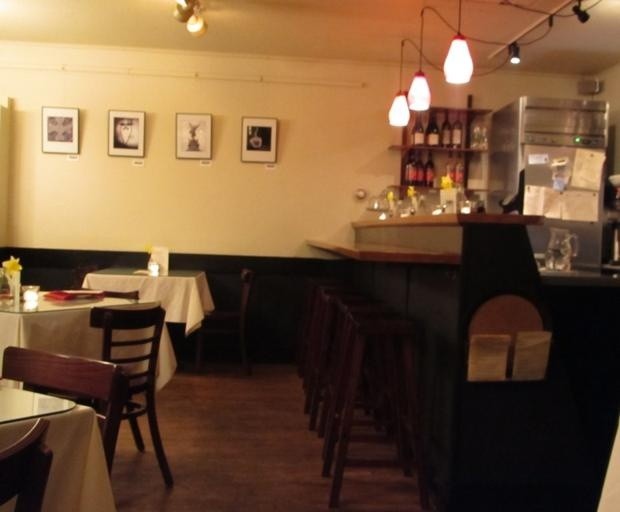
[107,109,147,159]
[174,112,213,161]
[240,116,279,164]
[40,105,80,155]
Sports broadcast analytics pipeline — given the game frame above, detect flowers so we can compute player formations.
[1,255,23,273]
[144,244,157,257]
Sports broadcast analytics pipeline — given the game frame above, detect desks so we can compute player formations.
[79,266,215,335]
[0,384,118,512]
[0,292,178,394]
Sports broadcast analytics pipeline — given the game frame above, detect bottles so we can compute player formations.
[365,106,494,218]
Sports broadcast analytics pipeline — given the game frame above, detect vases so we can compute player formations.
[6,271,22,300]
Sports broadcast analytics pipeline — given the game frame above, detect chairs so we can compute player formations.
[88,305,174,490]
[194,269,254,368]
[0,345,126,480]
[0,418,53,512]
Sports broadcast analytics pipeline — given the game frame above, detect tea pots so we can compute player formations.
[611,223,620,265]
[544,224,582,275]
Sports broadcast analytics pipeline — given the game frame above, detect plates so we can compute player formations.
[610,174,620,188]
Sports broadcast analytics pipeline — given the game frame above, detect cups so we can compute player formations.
[20,285,44,311]
[147,259,164,277]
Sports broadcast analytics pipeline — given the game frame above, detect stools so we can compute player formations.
[296,285,427,511]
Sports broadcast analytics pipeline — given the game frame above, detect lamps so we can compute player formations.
[173,0,207,34]
[386,1,602,127]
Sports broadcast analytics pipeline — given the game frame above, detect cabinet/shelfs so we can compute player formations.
[399,107,494,200]
